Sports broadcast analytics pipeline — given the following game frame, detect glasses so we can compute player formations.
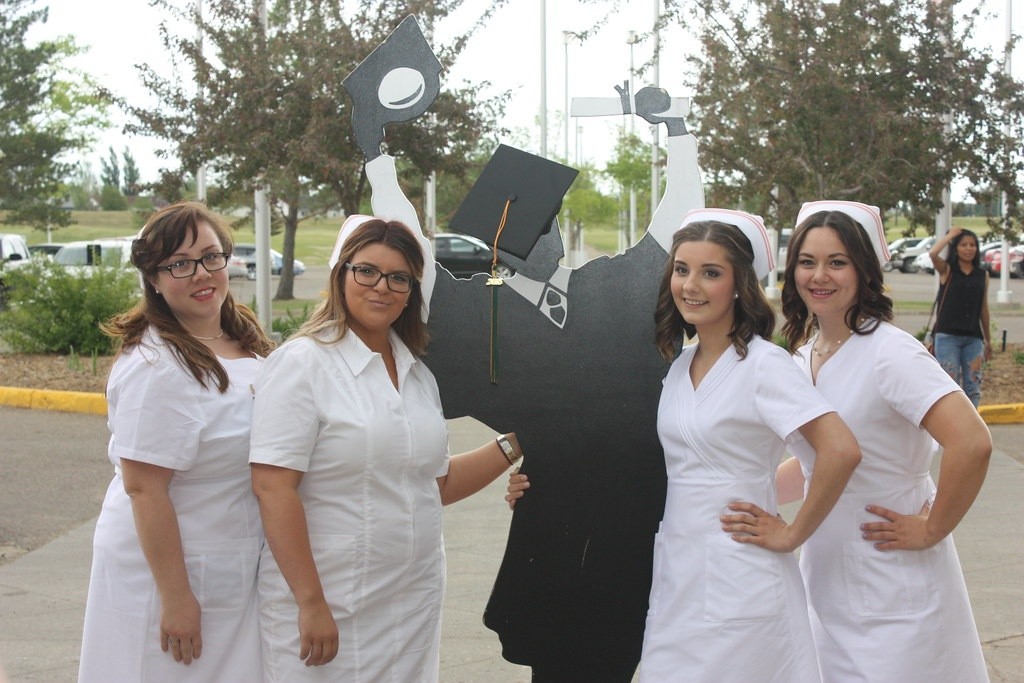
[152,250,230,279]
[344,261,415,293]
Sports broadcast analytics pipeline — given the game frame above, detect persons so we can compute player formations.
[506,206,860,683]
[928,226,993,411]
[76,199,276,683]
[773,200,1000,683]
[248,219,526,682]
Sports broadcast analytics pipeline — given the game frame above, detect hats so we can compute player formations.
[677,207,776,283]
[794,200,891,271]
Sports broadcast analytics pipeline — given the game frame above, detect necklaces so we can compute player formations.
[812,317,863,356]
[192,328,223,340]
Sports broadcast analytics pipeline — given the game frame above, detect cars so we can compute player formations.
[765,227,795,278]
[0,233,137,279]
[882,236,1024,279]
[232,244,306,277]
[435,233,515,279]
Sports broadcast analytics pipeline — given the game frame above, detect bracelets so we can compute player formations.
[497,434,520,464]
